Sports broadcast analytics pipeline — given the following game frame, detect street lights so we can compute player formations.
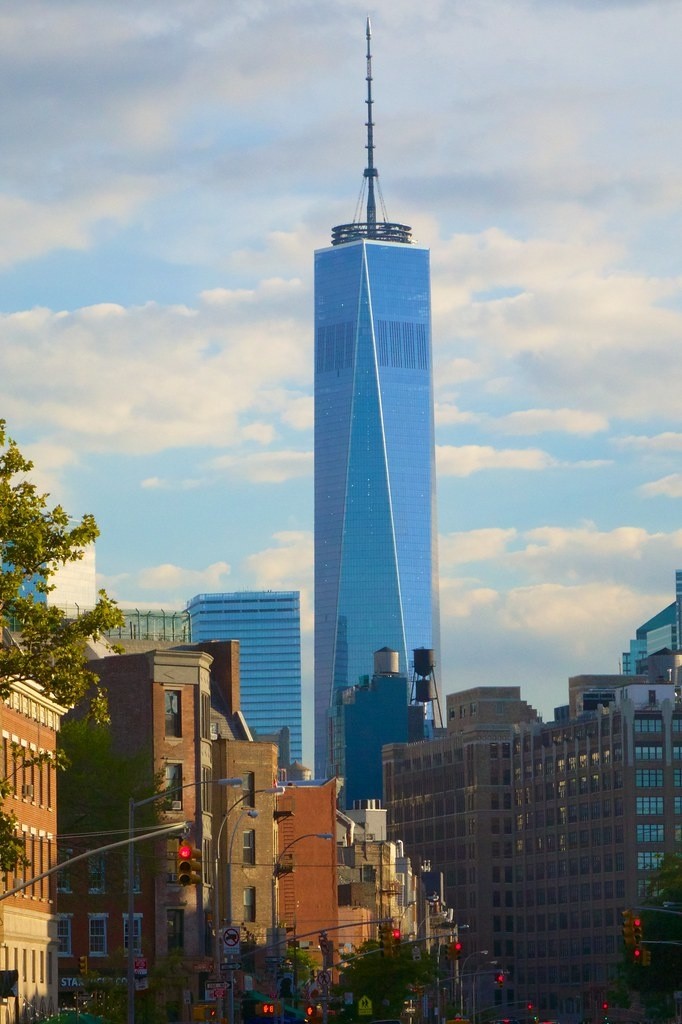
[458,950,489,1021]
[126,779,242,1024]
[399,896,469,1022]
[272,833,332,970]
[471,960,498,1023]
[211,786,285,1022]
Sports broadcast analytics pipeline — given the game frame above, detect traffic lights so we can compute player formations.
[643,950,651,967]
[634,919,642,943]
[193,846,202,886]
[378,924,400,958]
[496,975,503,990]
[79,956,88,976]
[635,949,641,957]
[178,845,193,886]
[306,1006,322,1017]
[534,1016,537,1019]
[620,910,632,944]
[445,941,462,960]
